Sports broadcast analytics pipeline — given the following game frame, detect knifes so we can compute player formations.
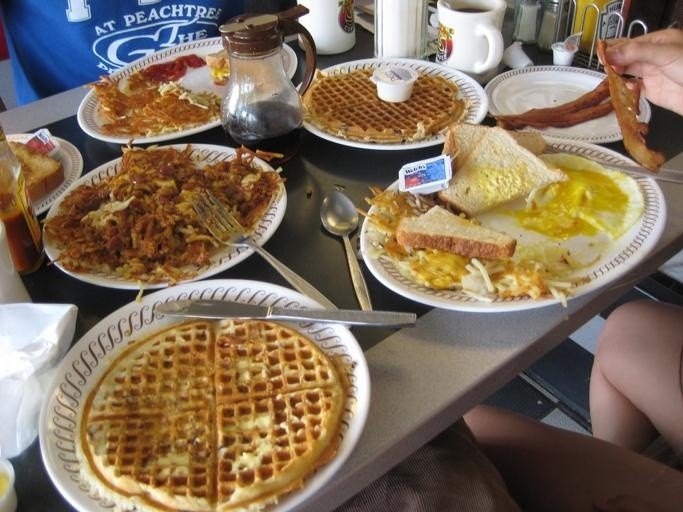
[155,301,416,327]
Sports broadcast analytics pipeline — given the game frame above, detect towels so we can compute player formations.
[0,302,78,460]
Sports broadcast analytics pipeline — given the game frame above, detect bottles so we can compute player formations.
[221,5,317,167]
[434,0,622,85]
[0,125,45,277]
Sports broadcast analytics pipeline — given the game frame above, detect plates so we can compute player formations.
[38,279,370,512]
[298,57,488,151]
[0,36,298,289]
[361,135,666,313]
[485,64,652,146]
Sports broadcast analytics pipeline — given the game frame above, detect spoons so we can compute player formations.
[322,190,371,311]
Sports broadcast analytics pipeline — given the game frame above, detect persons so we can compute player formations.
[333,416,523,512]
[462,27,683,512]
[0,0,245,107]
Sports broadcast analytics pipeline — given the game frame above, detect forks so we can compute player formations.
[191,189,352,329]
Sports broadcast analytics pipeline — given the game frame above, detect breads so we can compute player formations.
[301,69,466,144]
[80,318,345,510]
[393,127,569,261]
[0,140,62,212]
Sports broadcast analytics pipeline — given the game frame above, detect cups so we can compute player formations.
[296,0,429,65]
[0,459,18,510]
